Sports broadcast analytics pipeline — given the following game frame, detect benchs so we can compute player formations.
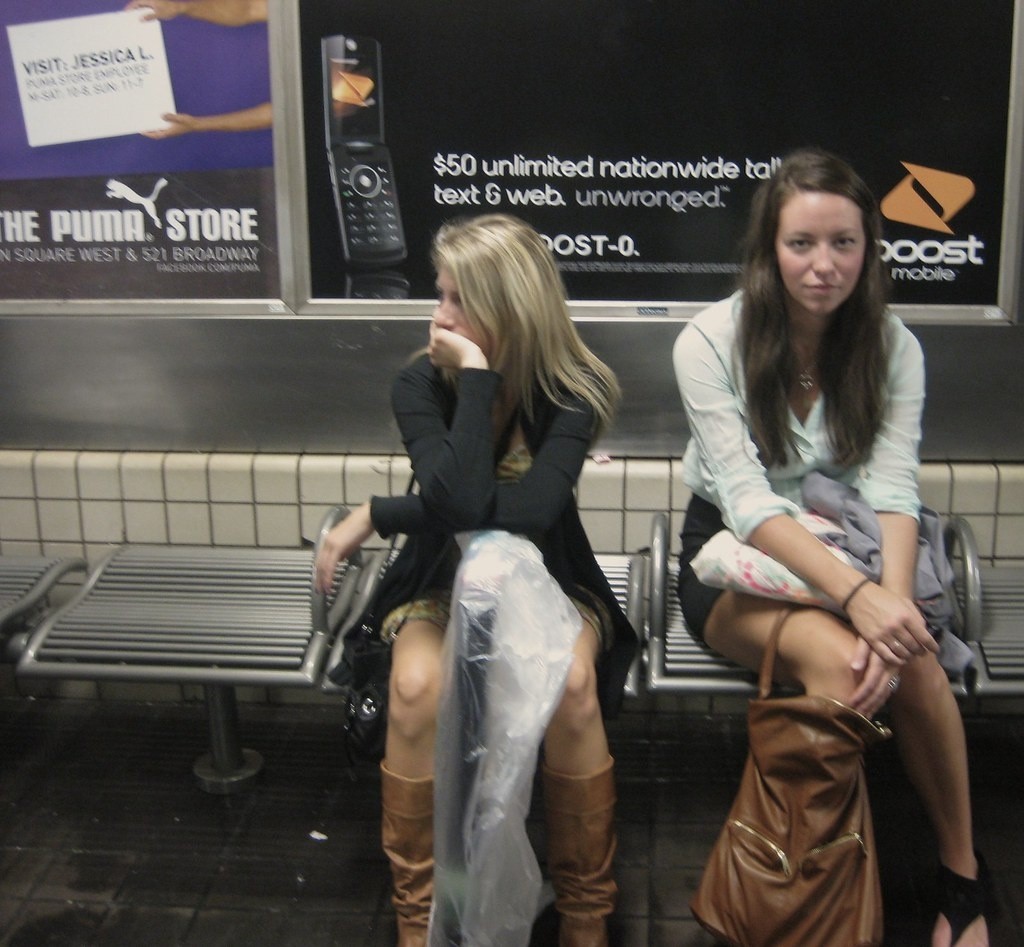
[0,508,1024,795]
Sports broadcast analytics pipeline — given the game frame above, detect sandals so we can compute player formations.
[928,846,996,947]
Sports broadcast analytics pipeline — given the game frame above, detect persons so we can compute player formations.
[313,214,641,947]
[670,150,1005,947]
[123,0,273,141]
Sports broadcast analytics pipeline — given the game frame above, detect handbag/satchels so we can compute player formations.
[688,497,856,623]
[346,639,392,760]
[689,601,894,947]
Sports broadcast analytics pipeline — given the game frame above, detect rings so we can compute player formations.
[888,675,901,692]
[889,640,900,651]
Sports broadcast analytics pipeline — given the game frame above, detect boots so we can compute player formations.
[540,754,618,947]
[380,758,435,947]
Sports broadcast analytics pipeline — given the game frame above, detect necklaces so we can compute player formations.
[791,345,821,391]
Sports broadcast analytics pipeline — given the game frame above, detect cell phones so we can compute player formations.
[320,34,407,263]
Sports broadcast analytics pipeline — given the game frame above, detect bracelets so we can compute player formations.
[841,578,871,609]
